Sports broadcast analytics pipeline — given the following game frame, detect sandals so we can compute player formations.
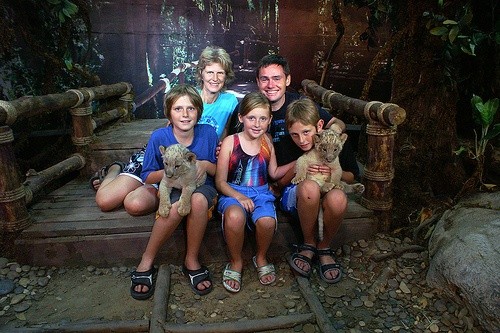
[88,167,109,191]
[182,263,218,295]
[129,264,157,300]
[316,247,341,283]
[289,244,317,277]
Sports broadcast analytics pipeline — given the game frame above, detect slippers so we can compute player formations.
[253,256,276,285]
[223,263,242,292]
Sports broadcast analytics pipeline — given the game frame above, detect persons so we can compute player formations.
[90,46,239,216]
[214,92,296,292]
[217,54,346,259]
[130,83,219,299]
[274,98,359,283]
